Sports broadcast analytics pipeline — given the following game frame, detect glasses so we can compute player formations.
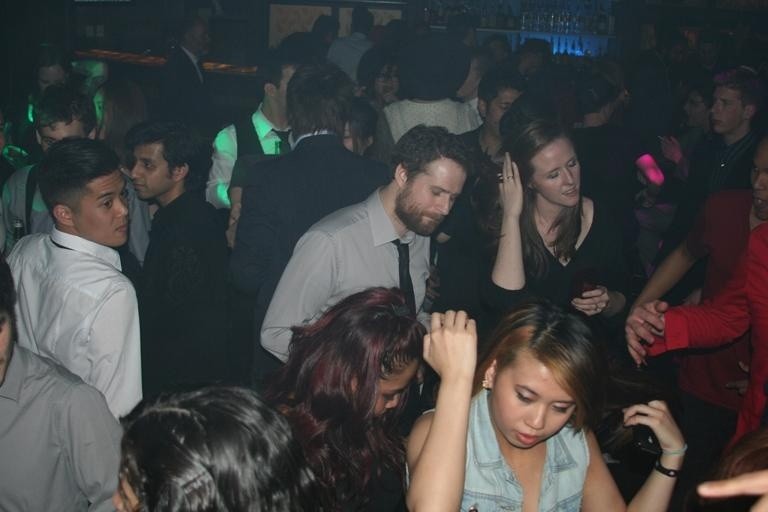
[376,72,400,83]
[683,96,705,108]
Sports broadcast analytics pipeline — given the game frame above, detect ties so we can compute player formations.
[270,128,292,155]
[392,238,416,323]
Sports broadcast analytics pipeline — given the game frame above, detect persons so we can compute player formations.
[0,1,767,512]
